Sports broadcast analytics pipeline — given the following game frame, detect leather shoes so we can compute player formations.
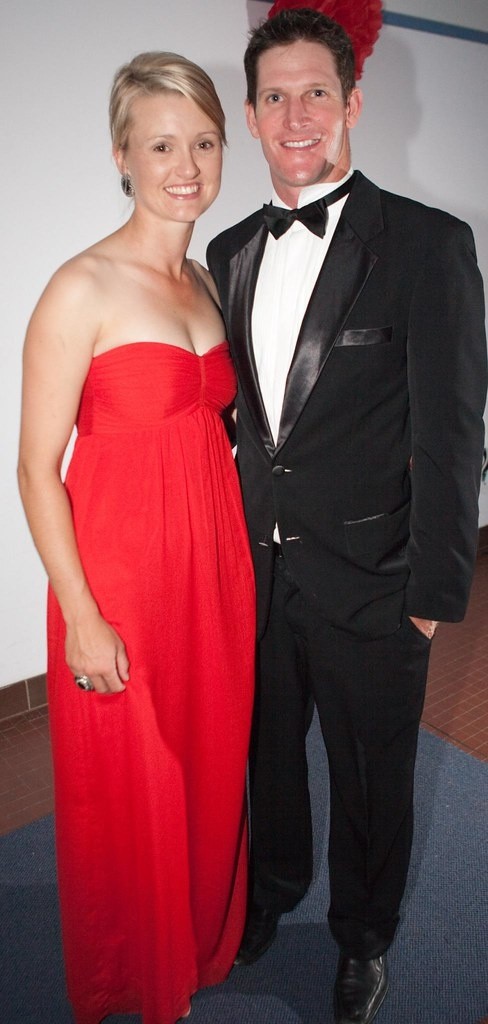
[334,951,389,1023]
[232,905,279,965]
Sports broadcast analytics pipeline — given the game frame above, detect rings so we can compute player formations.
[73,674,96,692]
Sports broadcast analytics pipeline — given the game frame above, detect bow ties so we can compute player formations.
[262,172,356,240]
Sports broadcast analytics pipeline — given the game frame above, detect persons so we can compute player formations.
[202,3,487,1024]
[14,46,263,1024]
